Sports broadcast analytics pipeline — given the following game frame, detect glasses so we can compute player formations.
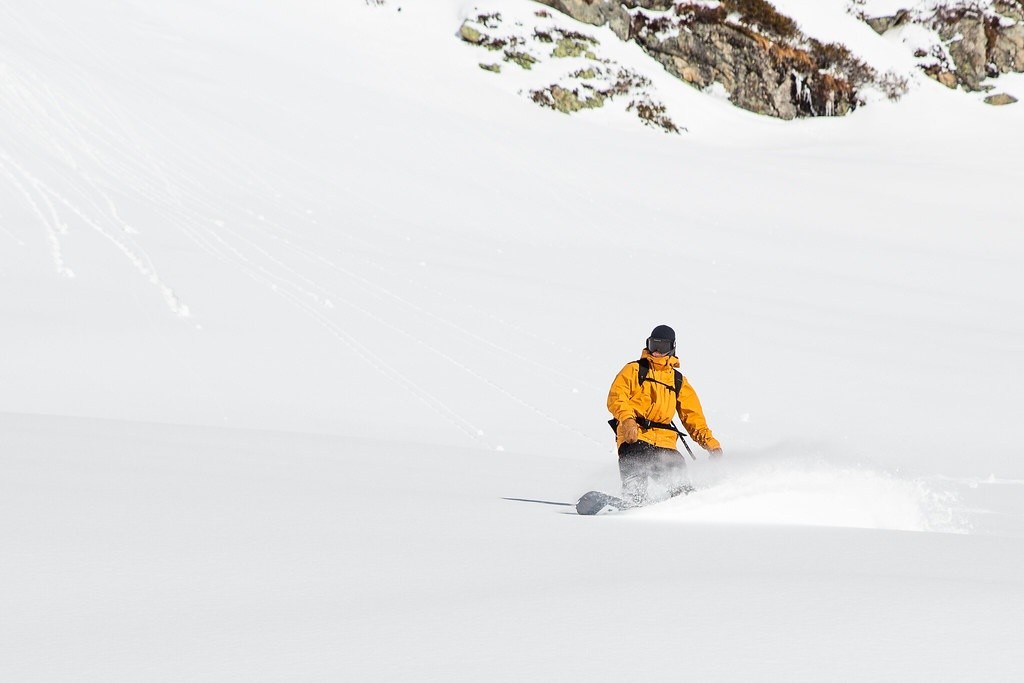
[649,339,671,354]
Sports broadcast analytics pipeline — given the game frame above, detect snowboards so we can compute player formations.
[574,490,645,516]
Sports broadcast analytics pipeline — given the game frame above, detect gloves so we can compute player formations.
[621,421,638,443]
[708,448,723,461]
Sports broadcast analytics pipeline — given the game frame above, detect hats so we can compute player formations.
[646,326,675,358]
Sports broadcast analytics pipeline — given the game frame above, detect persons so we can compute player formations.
[607,324,724,503]
